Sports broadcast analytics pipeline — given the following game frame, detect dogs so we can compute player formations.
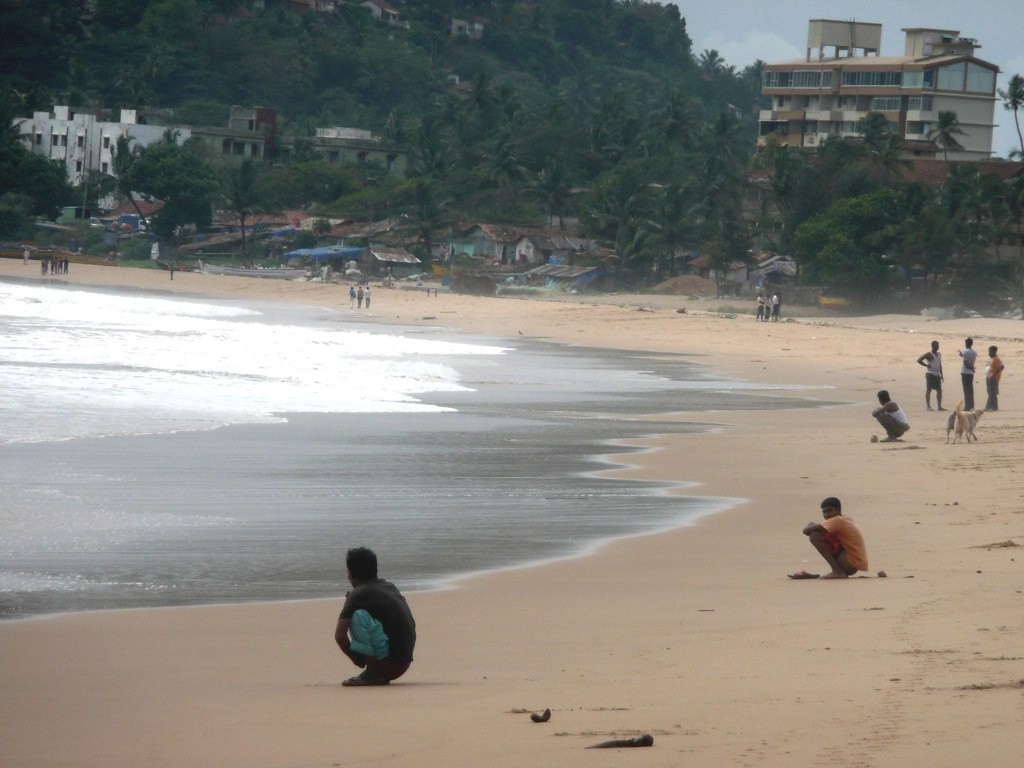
[945,401,984,443]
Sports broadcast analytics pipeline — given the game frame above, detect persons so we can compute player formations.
[349,286,372,309]
[24,248,69,275]
[872,389,910,442]
[335,547,417,686]
[917,340,948,411]
[958,338,977,411]
[802,496,869,580]
[982,345,1005,411]
[756,292,784,323]
[509,250,521,266]
